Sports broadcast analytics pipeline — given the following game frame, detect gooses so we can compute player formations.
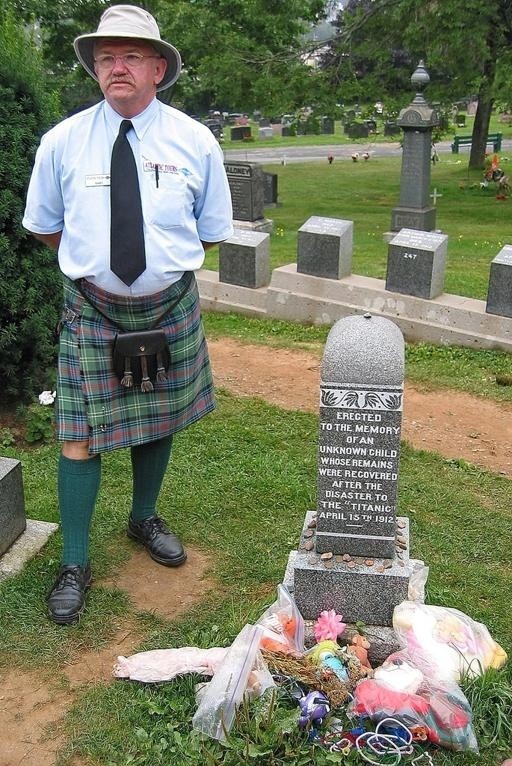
[362,153,369,161]
[352,153,359,162]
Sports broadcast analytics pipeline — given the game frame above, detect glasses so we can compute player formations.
[90,50,163,70]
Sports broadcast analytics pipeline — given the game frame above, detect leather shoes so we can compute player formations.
[47,560,92,626]
[126,510,187,568]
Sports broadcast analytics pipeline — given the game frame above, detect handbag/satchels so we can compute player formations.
[111,329,174,395]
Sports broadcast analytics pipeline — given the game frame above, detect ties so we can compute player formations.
[108,117,147,289]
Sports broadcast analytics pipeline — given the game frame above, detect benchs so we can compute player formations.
[448,131,503,157]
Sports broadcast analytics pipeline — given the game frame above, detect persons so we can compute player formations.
[327,150,334,163]
[363,152,369,160]
[480,169,509,195]
[20,4,234,620]
[352,152,359,161]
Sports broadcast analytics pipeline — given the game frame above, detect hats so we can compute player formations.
[74,5,181,94]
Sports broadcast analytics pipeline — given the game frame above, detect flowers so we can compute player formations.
[314,608,386,645]
